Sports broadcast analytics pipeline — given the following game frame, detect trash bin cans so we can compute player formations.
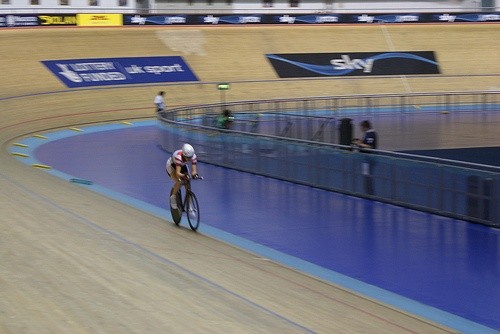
[338,118,354,145]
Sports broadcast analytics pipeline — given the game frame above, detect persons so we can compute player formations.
[216,110,234,133]
[352,120,378,195]
[154,91,165,112]
[166,143,199,219]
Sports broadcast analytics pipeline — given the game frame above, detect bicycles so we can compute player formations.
[169,174,204,231]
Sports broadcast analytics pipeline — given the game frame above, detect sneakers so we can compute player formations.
[184,206,195,218]
[170,194,178,210]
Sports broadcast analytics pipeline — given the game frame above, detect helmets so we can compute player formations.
[182,144,195,157]
